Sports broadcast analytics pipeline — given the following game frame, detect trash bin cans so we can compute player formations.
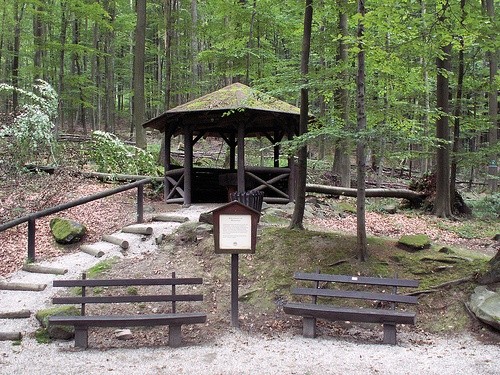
[229,190,265,224]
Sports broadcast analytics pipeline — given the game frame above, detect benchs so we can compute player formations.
[48,273,207,349]
[283,267,421,346]
